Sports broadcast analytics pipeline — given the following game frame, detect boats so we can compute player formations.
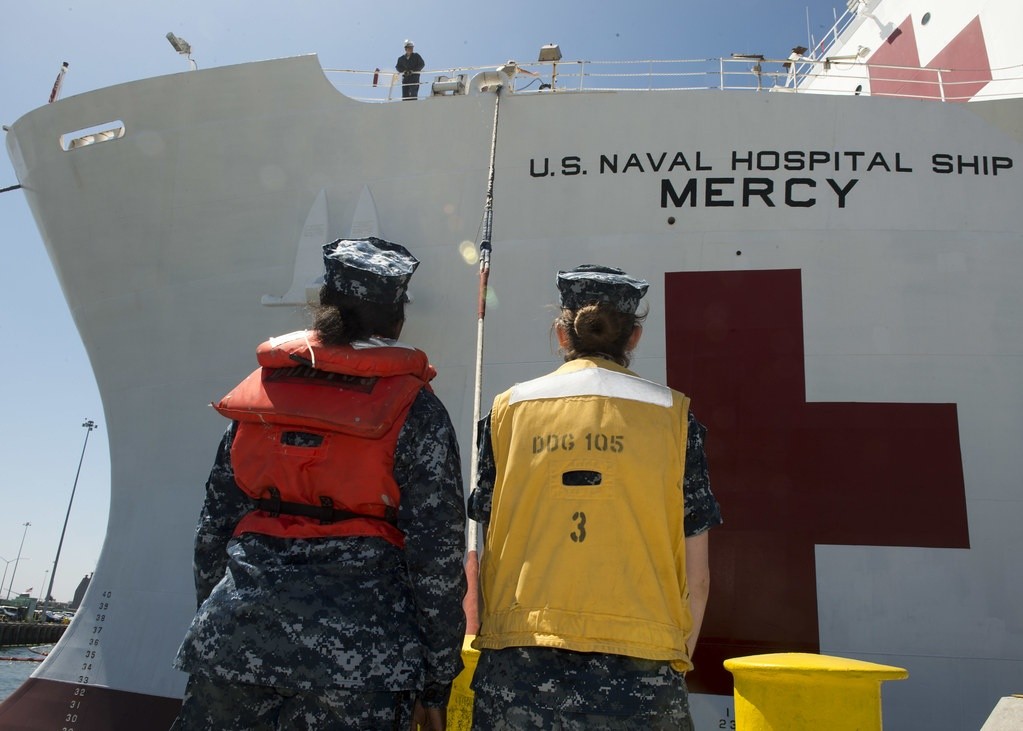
[0,1,1023,731]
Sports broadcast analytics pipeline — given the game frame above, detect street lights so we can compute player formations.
[36,569,50,605]
[4,521,34,608]
[40,419,100,624]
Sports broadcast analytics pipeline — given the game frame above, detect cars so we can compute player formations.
[0,603,78,625]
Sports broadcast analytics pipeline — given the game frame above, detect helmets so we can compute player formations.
[404,42,415,47]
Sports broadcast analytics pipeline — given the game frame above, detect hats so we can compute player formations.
[322,236,421,304]
[556,263,650,315]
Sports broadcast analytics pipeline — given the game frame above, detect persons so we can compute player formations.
[466,265,721,731]
[169,238,467,731]
[396,40,424,101]
